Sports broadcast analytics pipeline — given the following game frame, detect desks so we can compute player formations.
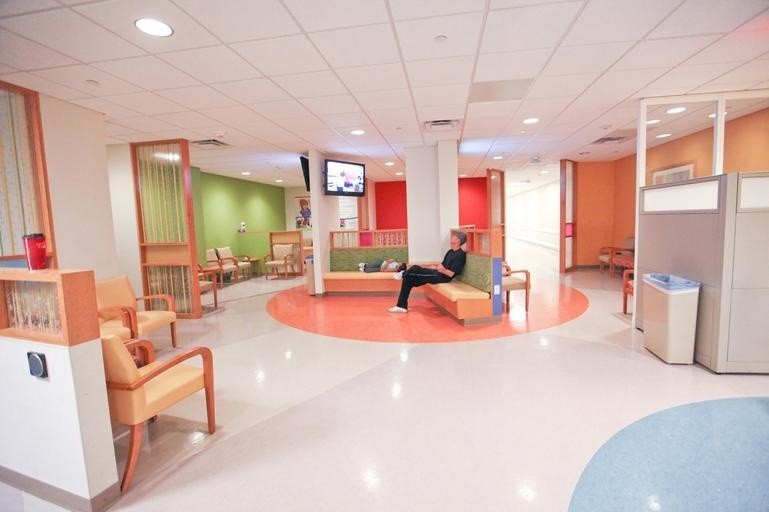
[242,258,262,276]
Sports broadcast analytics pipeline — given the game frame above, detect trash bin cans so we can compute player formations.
[304,255,315,295]
[643,274,701,364]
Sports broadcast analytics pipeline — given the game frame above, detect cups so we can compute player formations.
[21,232,48,271]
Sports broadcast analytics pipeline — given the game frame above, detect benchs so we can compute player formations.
[324,228,502,319]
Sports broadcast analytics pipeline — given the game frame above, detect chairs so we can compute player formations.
[502,263,530,311]
[264,244,298,279]
[599,247,634,314]
[101,334,215,495]
[94,274,176,367]
[197,247,251,307]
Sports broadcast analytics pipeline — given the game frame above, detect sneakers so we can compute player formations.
[389,306,408,313]
[359,263,368,271]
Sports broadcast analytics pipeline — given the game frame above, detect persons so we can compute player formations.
[299,198,311,218]
[353,175,362,192]
[388,228,467,313]
[359,258,406,272]
[337,172,345,192]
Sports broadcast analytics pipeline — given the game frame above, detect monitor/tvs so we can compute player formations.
[300,156,308,190]
[325,159,365,196]
